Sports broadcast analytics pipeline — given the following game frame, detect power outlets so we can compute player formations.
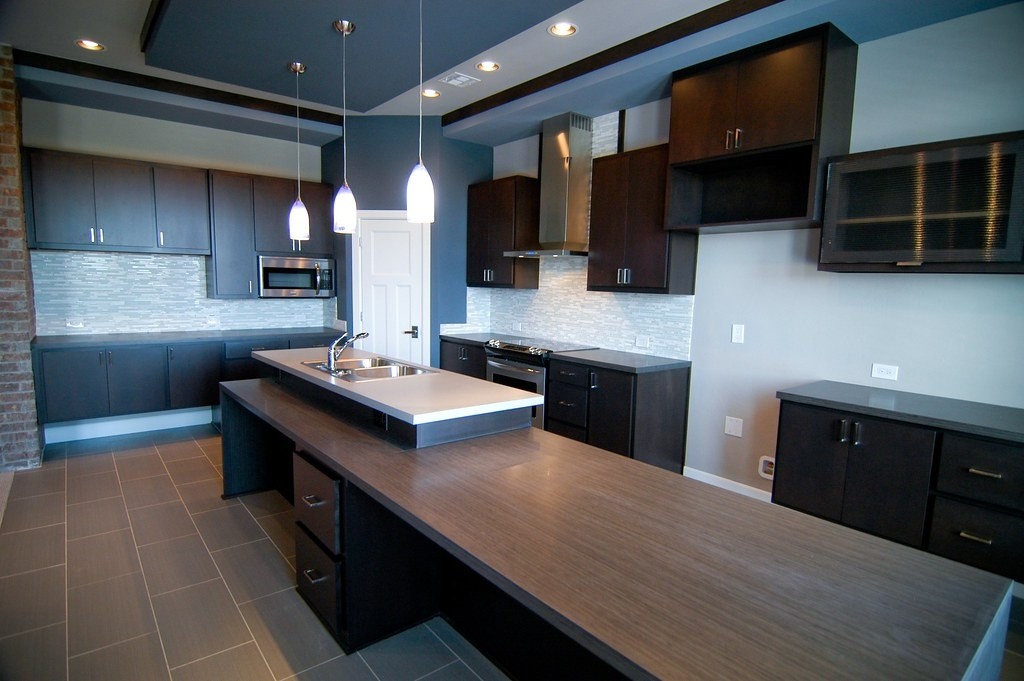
[871,363,898,380]
[731,325,744,343]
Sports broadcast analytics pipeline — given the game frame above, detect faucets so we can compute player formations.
[321,332,370,376]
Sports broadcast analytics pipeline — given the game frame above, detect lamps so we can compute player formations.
[287,61,309,240]
[332,20,357,234]
[406,0,435,223]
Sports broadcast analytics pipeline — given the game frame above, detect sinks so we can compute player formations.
[300,356,402,370]
[327,362,441,384]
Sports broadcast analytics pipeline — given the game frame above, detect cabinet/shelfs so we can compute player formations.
[586,143,699,296]
[663,22,859,236]
[292,449,353,657]
[925,429,1024,586]
[36,336,347,420]
[24,147,335,301]
[545,358,637,460]
[467,175,539,290]
[770,399,939,553]
[439,341,487,380]
